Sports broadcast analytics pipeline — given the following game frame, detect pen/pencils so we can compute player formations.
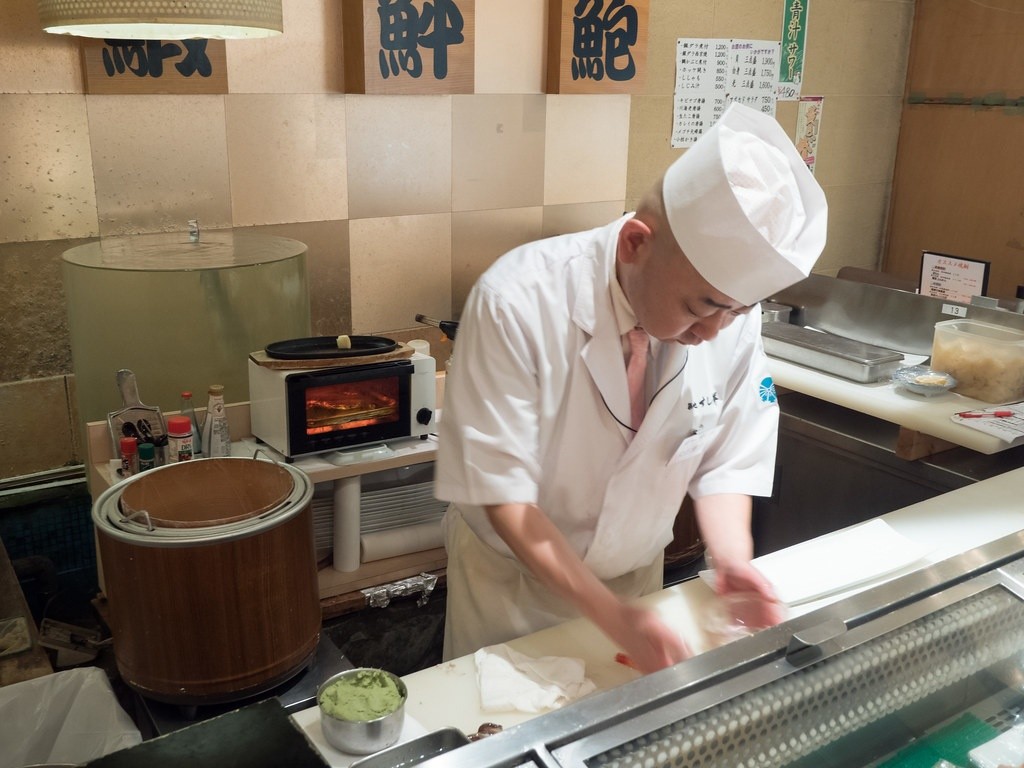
[958,411,1017,420]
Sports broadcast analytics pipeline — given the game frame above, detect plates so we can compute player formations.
[896,365,960,398]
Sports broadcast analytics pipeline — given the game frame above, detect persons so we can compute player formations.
[433,101,829,677]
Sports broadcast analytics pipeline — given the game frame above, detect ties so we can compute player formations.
[627,329,650,438]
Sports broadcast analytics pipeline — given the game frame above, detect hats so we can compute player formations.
[662,101,829,307]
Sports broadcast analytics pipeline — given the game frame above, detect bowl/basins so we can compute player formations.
[316,667,408,756]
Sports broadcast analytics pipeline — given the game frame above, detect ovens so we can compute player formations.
[248,351,439,464]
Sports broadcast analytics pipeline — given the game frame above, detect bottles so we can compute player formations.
[414,313,459,341]
[119,437,137,477]
[138,442,155,472]
[180,390,202,454]
[166,415,195,463]
[200,385,232,457]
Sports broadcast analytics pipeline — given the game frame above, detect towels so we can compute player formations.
[471,640,598,716]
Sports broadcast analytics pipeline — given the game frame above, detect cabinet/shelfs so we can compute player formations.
[85,370,449,634]
